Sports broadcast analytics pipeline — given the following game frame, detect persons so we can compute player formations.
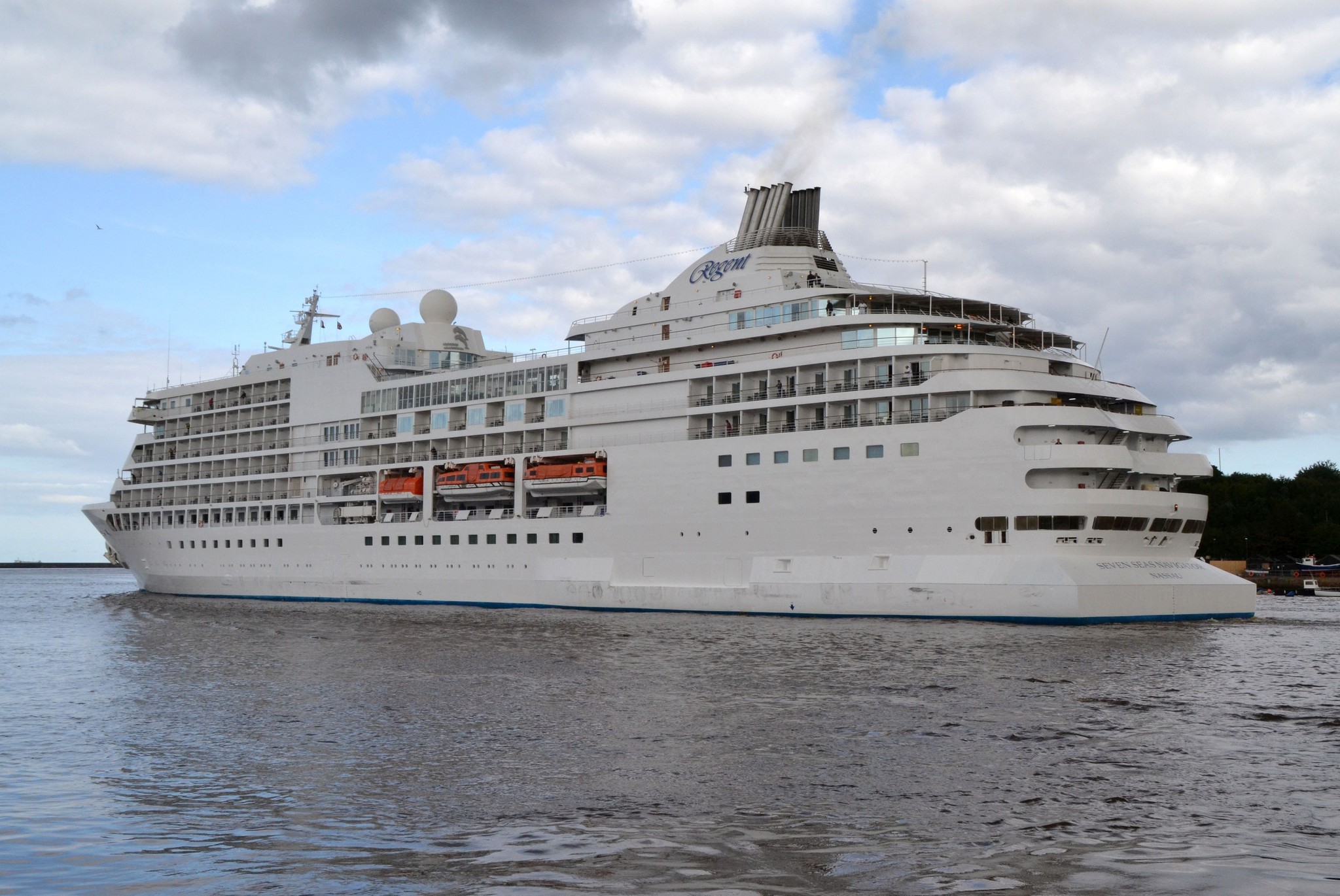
[156,493,162,506]
[807,271,816,288]
[209,398,213,409]
[131,473,136,484]
[169,446,176,459]
[726,420,731,437]
[858,300,868,314]
[462,388,466,401]
[904,366,913,386]
[227,489,231,502]
[158,471,163,481]
[431,446,437,460]
[814,273,821,288]
[650,358,668,373]
[240,390,246,405]
[826,300,833,316]
[186,422,190,429]
[790,281,801,289]
[776,380,782,396]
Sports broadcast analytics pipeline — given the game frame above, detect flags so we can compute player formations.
[320,320,325,328]
[337,321,342,329]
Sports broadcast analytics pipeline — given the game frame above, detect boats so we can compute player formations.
[1244,568,1269,574]
[435,463,516,503]
[1295,554,1340,575]
[378,472,423,504]
[1302,579,1340,597]
[522,458,606,498]
[77,180,1255,621]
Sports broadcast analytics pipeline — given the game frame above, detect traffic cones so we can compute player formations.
[1262,572,1265,577]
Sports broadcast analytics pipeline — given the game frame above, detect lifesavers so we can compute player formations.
[595,376,602,381]
[199,521,203,528]
[663,328,668,333]
[771,353,778,359]
[542,354,547,358]
[353,353,358,361]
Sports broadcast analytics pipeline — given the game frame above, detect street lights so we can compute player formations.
[1213,539,1216,558]
[1245,538,1249,563]
